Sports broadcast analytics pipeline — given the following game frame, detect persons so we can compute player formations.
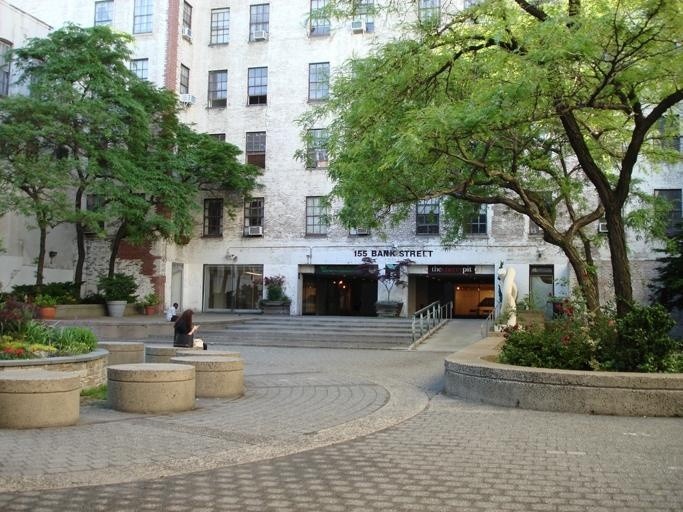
[165,302,180,322]
[498,266,517,328]
[173,309,209,350]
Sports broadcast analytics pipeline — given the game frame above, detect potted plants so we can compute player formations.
[96,271,162,317]
[352,256,416,318]
[33,294,57,320]
[251,276,292,315]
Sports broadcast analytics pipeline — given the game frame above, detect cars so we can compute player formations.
[479,297,494,316]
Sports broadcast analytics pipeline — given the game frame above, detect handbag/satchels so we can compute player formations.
[174,333,193,348]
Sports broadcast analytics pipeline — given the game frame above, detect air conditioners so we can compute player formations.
[351,20,367,34]
[180,93,196,105]
[182,28,193,39]
[355,226,370,235]
[253,30,267,41]
[247,225,263,236]
[598,222,608,233]
